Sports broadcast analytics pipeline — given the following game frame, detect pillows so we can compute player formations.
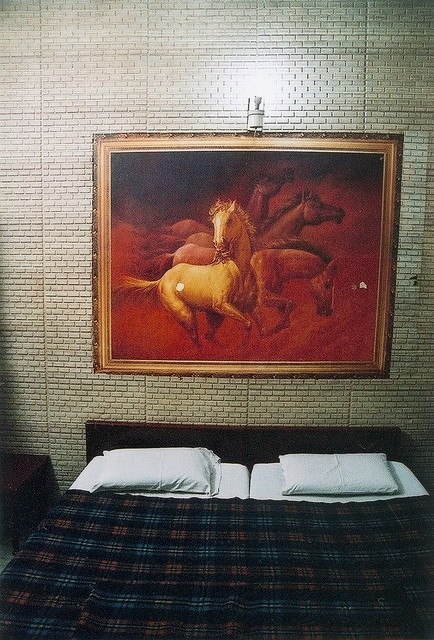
[277,453,399,495]
[90,447,222,495]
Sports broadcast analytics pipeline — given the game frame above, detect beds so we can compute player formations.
[0,419,434,640]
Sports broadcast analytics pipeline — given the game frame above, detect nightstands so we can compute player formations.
[0,453,49,555]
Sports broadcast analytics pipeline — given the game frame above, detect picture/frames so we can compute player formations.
[92,132,403,378]
